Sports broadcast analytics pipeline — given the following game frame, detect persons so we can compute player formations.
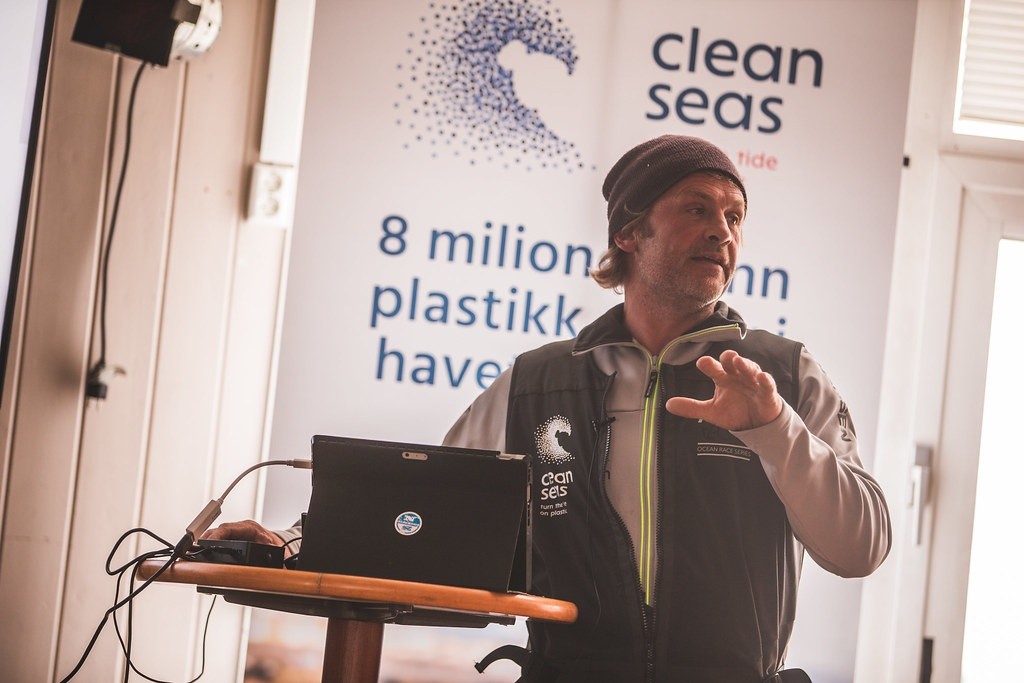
[194,134,894,683]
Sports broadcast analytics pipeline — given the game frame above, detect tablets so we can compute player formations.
[308,435,535,593]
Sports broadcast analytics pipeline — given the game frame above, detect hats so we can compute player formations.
[601,134,747,251]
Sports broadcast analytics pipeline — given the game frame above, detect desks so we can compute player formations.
[135,558,579,683]
